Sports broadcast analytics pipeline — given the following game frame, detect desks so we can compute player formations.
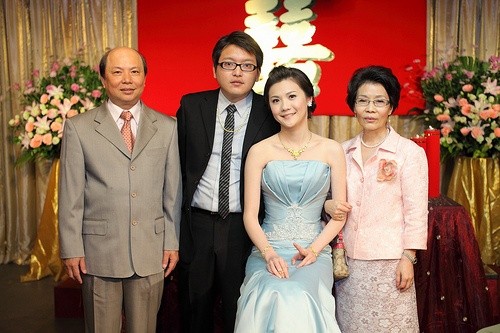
[413,196,496,333]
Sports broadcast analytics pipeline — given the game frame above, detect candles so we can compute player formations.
[410,135,427,150]
[424,126,441,199]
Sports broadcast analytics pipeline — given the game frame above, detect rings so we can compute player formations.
[278,269,282,271]
[412,282,413,284]
[341,216,342,218]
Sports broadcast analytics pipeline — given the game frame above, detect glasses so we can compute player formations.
[217,61,257,72]
[355,98,390,107]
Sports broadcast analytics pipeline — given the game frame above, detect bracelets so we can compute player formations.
[261,245,270,256]
[309,246,318,257]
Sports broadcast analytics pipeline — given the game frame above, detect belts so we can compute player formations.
[190,207,243,220]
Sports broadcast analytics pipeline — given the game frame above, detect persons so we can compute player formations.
[175,28,281,333]
[58,47,183,333]
[233,65,348,333]
[321,65,429,333]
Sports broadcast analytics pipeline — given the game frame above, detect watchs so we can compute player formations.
[402,251,417,264]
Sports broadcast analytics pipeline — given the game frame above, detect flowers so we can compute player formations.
[376,159,397,183]
[8,57,106,167]
[407,52,500,161]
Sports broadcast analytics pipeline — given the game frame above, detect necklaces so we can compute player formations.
[278,131,313,160]
[361,134,386,148]
[217,109,249,133]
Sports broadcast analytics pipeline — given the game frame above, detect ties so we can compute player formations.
[218,104,237,220]
[120,111,134,154]
